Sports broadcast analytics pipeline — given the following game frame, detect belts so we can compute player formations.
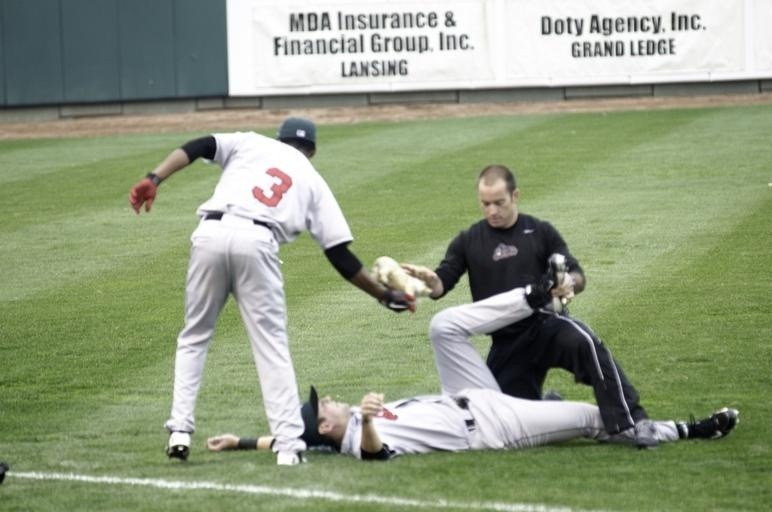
[206,214,272,229]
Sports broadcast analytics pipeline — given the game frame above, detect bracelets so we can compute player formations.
[240,433,262,453]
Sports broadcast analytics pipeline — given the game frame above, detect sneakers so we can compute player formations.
[277,452,307,465]
[526,252,564,314]
[686,408,740,438]
[635,419,659,449]
[166,430,191,459]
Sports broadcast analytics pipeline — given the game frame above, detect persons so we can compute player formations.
[205,249,737,459]
[128,116,419,464]
[399,164,681,444]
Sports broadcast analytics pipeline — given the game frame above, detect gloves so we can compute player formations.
[130,172,161,213]
[299,386,322,447]
[380,284,416,312]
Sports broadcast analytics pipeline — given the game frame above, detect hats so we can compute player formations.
[280,118,316,143]
